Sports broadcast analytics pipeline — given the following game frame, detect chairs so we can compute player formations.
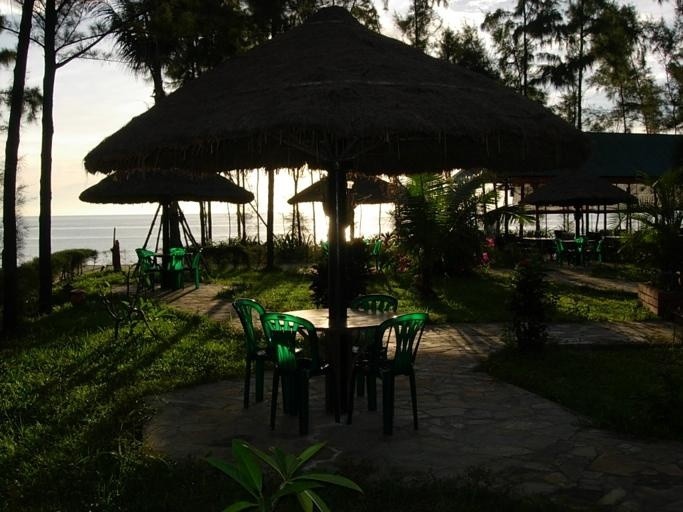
[320,239,385,276]
[553,230,609,271]
[232,292,429,436]
[133,243,204,295]
